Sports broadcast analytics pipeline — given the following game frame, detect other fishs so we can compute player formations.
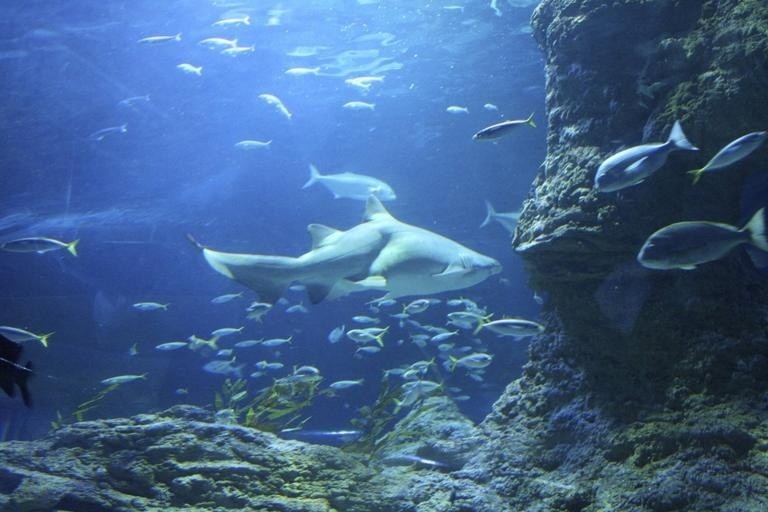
[88,0,546,422]
